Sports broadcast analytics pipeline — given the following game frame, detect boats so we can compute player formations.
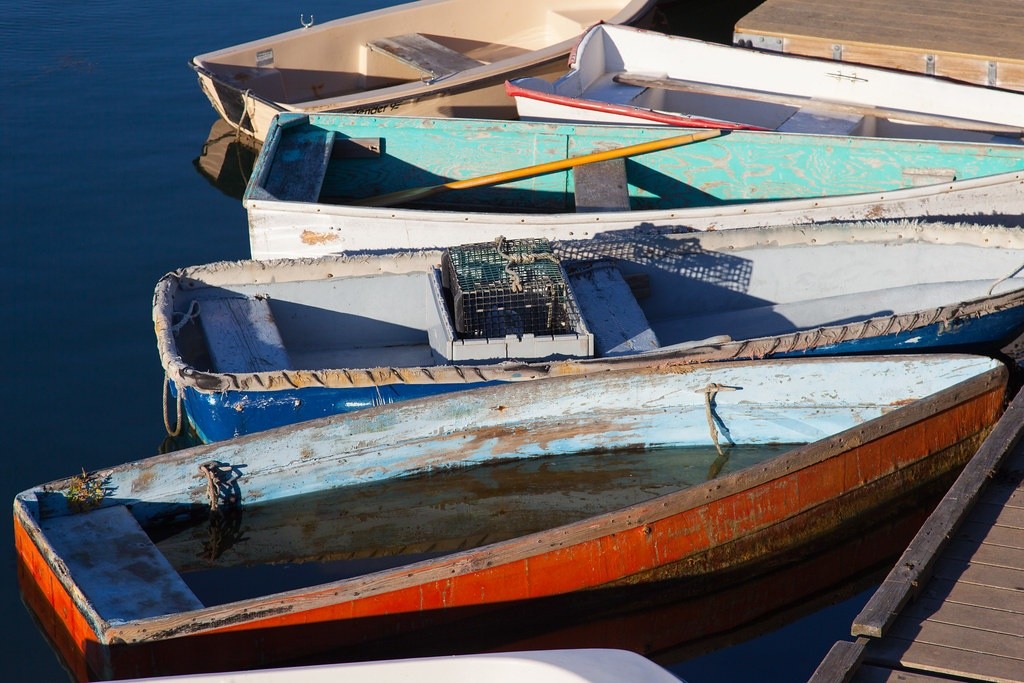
[152,221,1024,445]
[104,647,682,682]
[732,0,1024,96]
[243,110,1024,261]
[504,19,1024,146]
[184,0,661,141]
[11,352,1012,683]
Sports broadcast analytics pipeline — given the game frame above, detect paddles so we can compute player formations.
[505,81,748,131]
[361,127,723,206]
